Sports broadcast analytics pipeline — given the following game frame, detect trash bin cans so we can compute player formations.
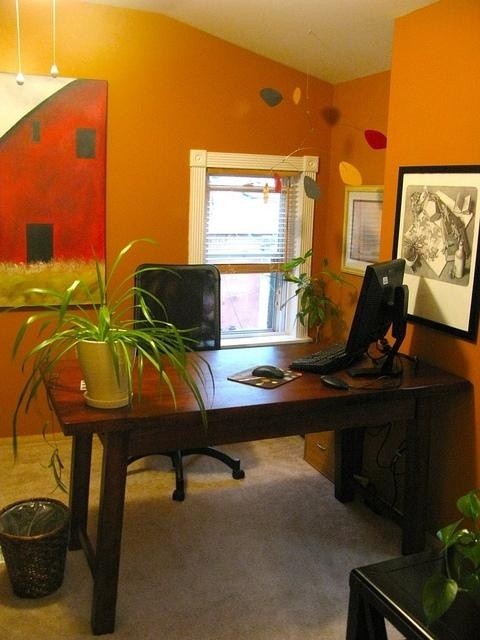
[0,497,70,599]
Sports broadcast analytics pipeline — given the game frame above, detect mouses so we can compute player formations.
[252,365,284,378]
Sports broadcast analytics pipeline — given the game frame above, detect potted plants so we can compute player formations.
[7,238,215,489]
[278,246,358,341]
[405,489,480,633]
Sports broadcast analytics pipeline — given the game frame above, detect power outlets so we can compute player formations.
[402,457,425,479]
[378,429,396,448]
[359,484,389,504]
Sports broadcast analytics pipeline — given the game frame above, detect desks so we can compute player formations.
[38,348,470,634]
[345,548,480,640]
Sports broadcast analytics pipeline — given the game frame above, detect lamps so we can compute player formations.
[16,2,25,86]
[50,14,58,78]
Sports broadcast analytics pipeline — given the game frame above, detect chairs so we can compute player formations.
[129,263,245,500]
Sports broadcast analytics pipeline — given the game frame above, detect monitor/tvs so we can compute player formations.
[345,258,409,378]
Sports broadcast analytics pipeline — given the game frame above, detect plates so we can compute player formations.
[83,390,133,409]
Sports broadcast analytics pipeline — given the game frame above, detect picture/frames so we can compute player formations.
[341,186,385,278]
[388,165,480,341]
[0,74,108,308]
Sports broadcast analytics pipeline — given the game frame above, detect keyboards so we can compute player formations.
[288,342,347,375]
